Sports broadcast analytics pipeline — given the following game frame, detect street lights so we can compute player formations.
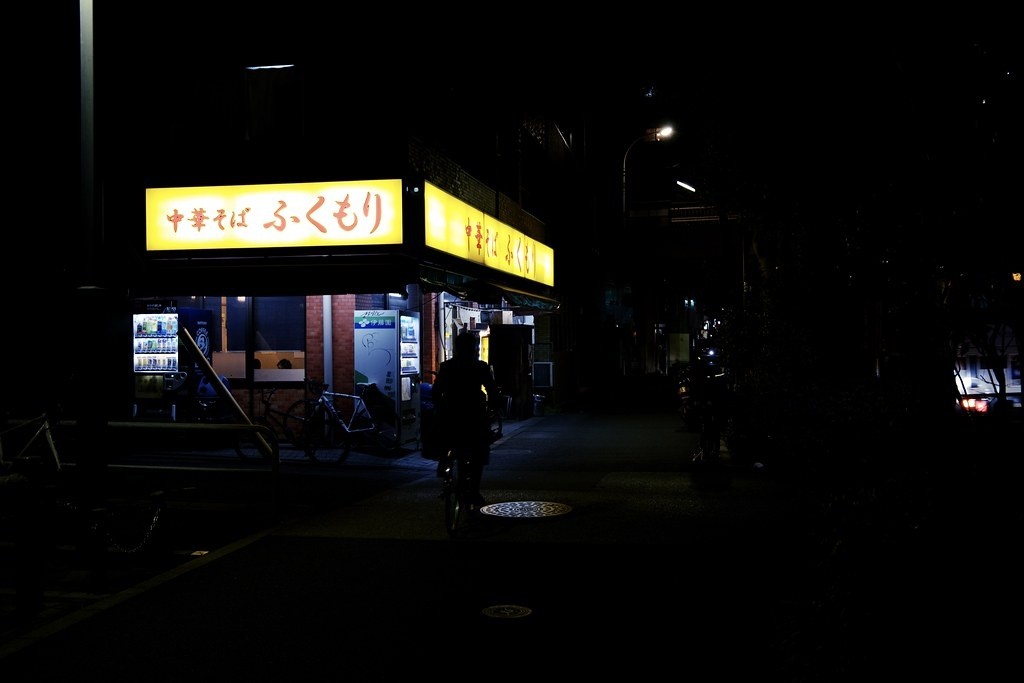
[615,121,679,414]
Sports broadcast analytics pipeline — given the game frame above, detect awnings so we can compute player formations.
[110,257,413,301]
[417,269,560,317]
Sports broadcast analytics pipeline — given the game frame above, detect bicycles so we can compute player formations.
[434,401,508,535]
[233,381,350,466]
[281,373,404,455]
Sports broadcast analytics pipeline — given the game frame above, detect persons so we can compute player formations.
[430,331,501,502]
